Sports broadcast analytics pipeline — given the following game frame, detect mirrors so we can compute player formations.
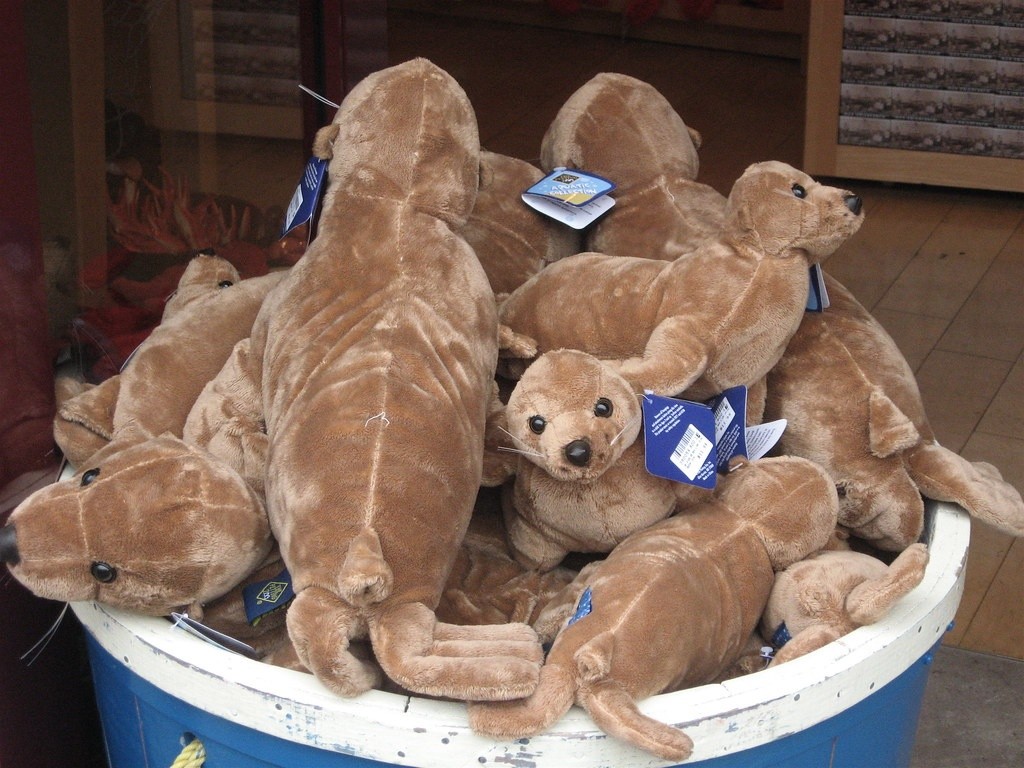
[65,0,344,318]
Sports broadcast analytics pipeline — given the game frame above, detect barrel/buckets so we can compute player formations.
[66,501,971,768]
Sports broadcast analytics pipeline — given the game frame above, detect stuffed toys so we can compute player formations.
[0,57,1024,761]
